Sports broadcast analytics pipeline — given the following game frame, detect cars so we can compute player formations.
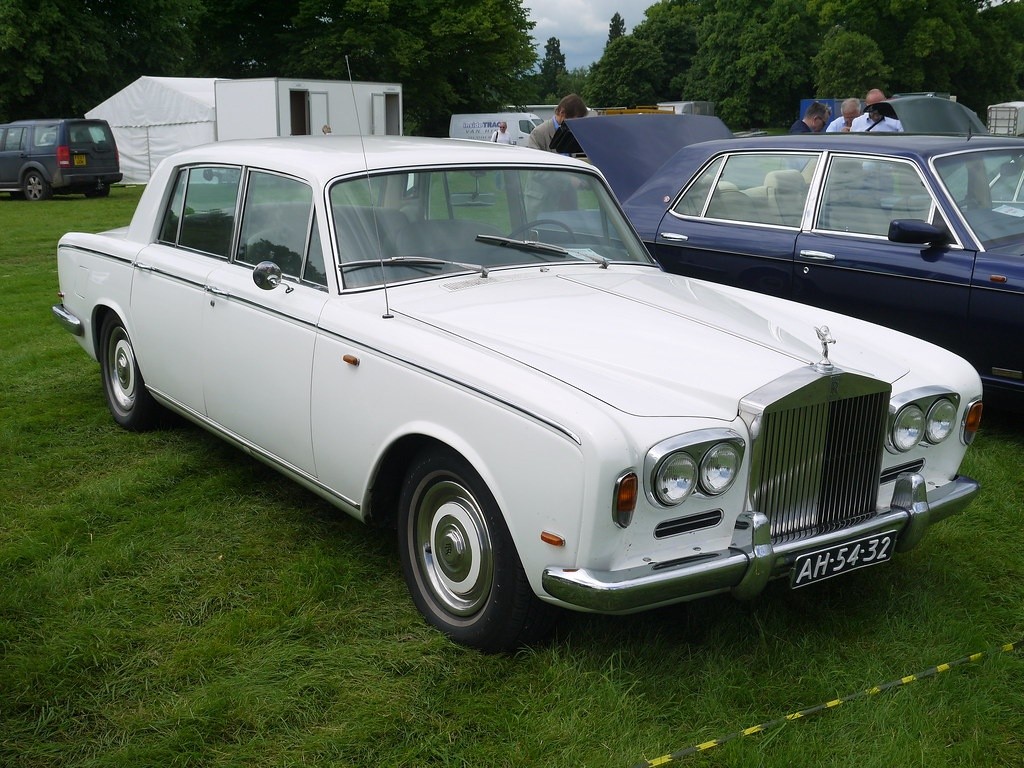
[868,96,1024,214]
[539,110,1024,417]
[52,133,986,656]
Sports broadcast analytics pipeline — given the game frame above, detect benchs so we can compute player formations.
[171,203,408,252]
[688,168,808,227]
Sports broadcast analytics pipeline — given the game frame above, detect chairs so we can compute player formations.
[893,171,930,220]
[246,225,319,274]
[826,160,891,234]
[392,220,505,258]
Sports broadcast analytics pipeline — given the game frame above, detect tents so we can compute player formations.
[84,75,229,186]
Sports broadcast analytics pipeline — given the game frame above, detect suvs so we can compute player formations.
[0,118,123,202]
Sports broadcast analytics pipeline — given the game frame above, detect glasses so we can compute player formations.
[819,117,826,127]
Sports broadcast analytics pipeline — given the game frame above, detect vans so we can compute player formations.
[449,114,543,147]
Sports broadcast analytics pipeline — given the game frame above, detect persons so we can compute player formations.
[787,102,830,171]
[491,122,512,190]
[848,88,904,199]
[525,94,588,221]
[826,98,862,132]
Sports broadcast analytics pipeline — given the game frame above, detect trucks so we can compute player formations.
[657,101,715,116]
